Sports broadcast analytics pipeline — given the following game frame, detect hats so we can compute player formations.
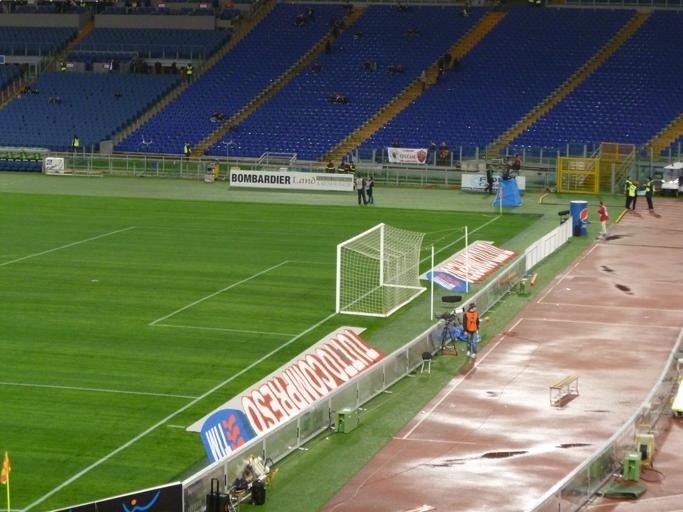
[469,303,476,311]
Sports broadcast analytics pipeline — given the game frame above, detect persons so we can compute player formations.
[0,0,259,124]
[364,176,375,204]
[354,174,369,204]
[642,176,654,212]
[505,153,521,179]
[290,1,541,108]
[327,159,335,173]
[484,164,496,194]
[596,199,609,235]
[70,134,80,152]
[627,183,638,210]
[338,160,346,173]
[438,141,449,163]
[180,139,192,161]
[344,161,356,173]
[426,142,436,164]
[461,302,479,359]
[624,175,632,207]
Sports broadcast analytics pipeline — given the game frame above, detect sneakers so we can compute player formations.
[471,354,476,358]
[467,351,471,356]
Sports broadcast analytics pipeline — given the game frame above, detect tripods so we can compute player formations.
[439,319,458,356]
